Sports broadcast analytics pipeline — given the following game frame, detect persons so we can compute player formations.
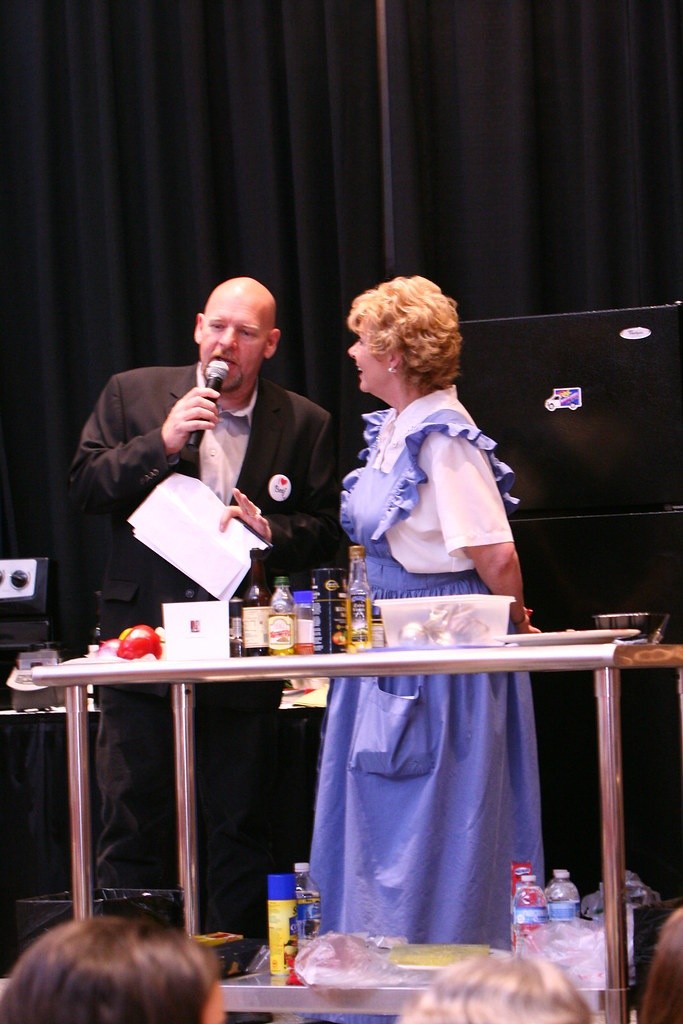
[293,275,542,1023]
[67,274,347,936]
[0,916,226,1024]
[396,951,594,1023]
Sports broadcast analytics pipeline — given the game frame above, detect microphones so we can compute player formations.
[186,361,229,450]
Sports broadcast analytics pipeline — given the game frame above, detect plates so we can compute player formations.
[395,948,513,973]
[497,628,640,646]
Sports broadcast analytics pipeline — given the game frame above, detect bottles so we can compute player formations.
[229,545,386,658]
[290,862,321,950]
[513,875,548,952]
[546,870,581,926]
[267,873,299,975]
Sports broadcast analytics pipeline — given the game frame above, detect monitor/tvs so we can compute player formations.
[454,302,683,523]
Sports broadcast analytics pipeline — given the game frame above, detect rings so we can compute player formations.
[247,505,261,518]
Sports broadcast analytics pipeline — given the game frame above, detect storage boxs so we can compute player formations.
[368,594,519,649]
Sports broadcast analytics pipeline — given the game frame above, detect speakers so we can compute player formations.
[1,709,100,947]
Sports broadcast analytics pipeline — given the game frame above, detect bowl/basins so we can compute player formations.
[593,612,671,645]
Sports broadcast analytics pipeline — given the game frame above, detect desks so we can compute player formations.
[31,638,683,1023]
